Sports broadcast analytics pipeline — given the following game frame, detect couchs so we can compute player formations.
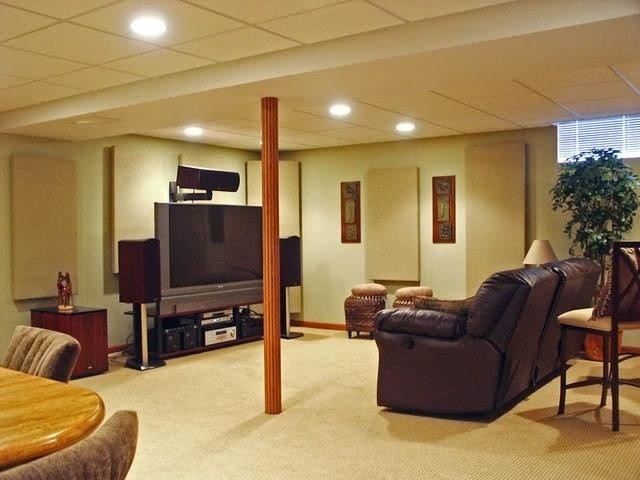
[375,258,602,423]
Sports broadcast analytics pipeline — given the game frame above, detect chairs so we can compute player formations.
[0,410,138,480]
[557,242,640,431]
[0,325,82,383]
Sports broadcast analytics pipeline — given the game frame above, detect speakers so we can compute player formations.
[175,164,240,193]
[281,235,303,288]
[117,238,161,305]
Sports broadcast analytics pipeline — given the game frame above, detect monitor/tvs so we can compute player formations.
[152,201,263,298]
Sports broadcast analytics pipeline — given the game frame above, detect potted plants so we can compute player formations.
[550,147,640,363]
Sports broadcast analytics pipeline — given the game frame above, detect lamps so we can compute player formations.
[523,239,557,267]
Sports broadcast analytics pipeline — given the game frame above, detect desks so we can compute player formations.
[0,366,105,468]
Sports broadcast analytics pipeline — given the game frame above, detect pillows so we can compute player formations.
[395,287,432,305]
[411,294,467,313]
[352,283,387,300]
[590,248,611,319]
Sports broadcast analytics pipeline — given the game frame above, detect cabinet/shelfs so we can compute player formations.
[126,300,264,360]
[31,306,108,380]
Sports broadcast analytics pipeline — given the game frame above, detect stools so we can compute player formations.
[345,296,385,339]
[394,302,416,309]
